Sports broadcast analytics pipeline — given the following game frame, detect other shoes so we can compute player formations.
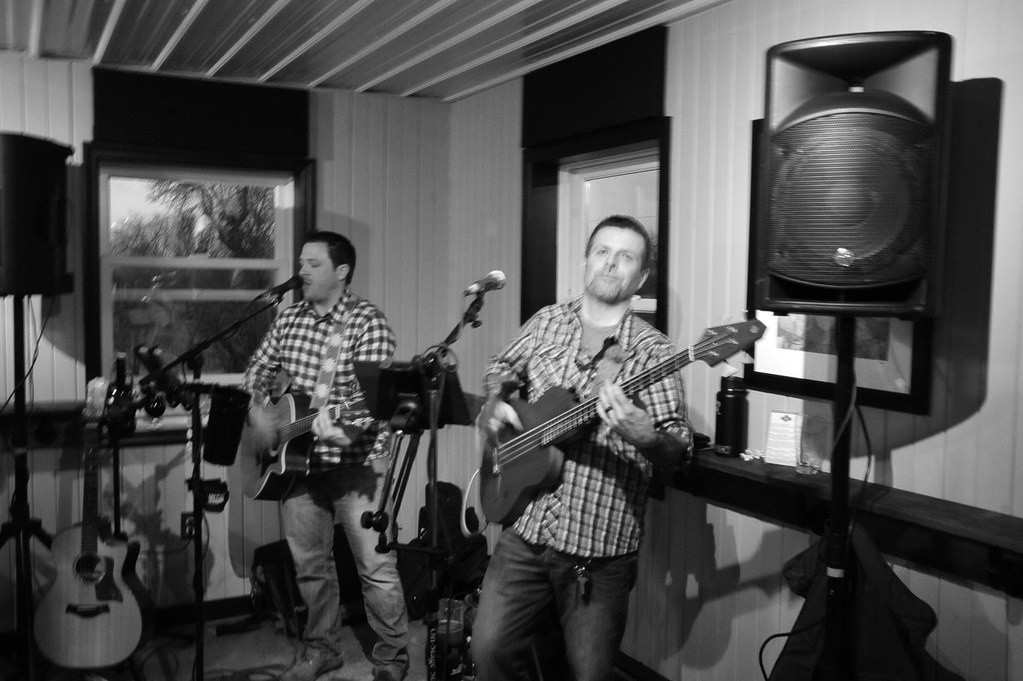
[279,651,343,681]
[372,667,405,681]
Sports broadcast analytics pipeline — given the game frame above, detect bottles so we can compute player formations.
[105,352,136,435]
[715,376,749,456]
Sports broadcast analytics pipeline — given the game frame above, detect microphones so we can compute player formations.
[257,275,305,299]
[464,270,506,296]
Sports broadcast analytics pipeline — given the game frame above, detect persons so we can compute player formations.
[241,231,409,681]
[473,214,694,681]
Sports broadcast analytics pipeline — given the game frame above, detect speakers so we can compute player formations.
[0,131,72,298]
[754,30,953,324]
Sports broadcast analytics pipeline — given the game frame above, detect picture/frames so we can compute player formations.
[746,117,936,415]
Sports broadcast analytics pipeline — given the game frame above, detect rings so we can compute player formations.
[605,406,613,414]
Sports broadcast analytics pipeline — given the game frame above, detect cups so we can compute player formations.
[464,594,478,637]
[795,412,828,475]
[436,598,464,643]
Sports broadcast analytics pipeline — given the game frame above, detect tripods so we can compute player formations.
[0,295,145,681]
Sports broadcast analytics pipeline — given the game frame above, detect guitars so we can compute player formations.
[31,374,145,671]
[234,389,372,502]
[478,316,768,527]
